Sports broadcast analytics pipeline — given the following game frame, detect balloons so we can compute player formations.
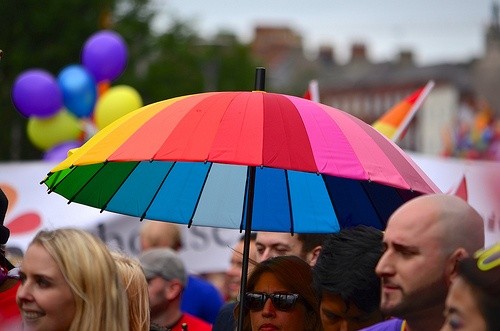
[12,32,142,162]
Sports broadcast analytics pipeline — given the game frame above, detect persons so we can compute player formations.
[0,187,499,331]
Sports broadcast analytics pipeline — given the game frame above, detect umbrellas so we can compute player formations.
[39,67,444,331]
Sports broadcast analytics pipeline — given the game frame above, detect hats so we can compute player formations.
[138,247,185,281]
[0,189,10,244]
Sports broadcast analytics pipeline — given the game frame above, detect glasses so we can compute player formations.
[244,291,315,314]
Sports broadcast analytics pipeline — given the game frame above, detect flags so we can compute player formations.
[370,80,435,146]
[448,173,468,203]
[303,79,319,102]
